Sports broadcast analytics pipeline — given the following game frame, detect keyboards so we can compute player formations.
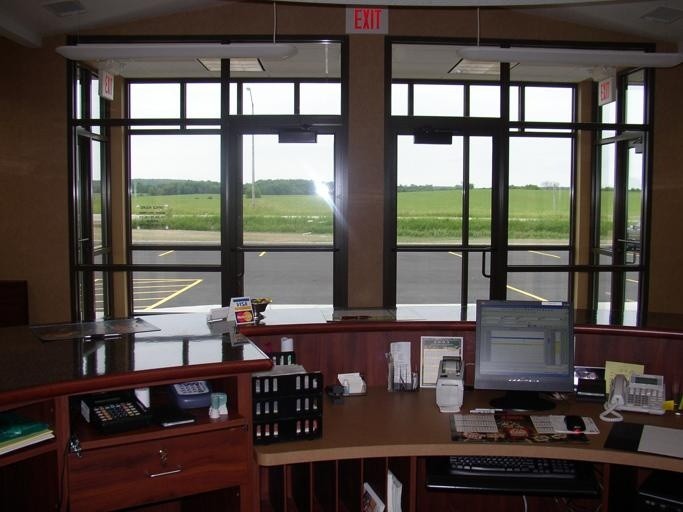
[446,456,580,484]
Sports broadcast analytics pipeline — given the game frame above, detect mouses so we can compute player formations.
[563,414,585,431]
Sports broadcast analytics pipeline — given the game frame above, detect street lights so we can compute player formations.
[245,86,255,208]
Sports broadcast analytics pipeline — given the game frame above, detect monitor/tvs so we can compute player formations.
[475,299,573,411]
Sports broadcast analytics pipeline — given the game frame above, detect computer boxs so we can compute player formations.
[637,475,683,512]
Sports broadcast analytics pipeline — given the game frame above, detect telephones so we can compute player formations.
[599,373,666,421]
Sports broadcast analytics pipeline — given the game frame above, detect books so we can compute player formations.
[362,482,386,512]
[388,471,402,511]
[0,422,55,455]
